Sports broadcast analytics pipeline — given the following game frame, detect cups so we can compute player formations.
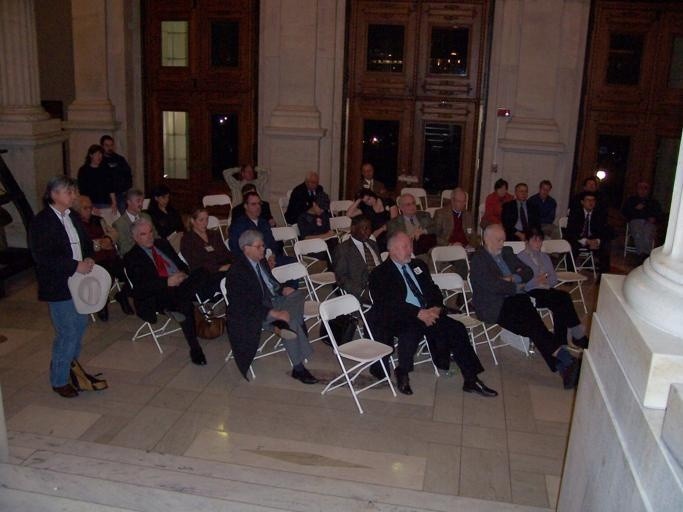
[92,240,100,252]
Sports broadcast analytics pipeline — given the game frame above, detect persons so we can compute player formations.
[72,136,287,319]
[0,204,11,343]
[368,227,499,398]
[286,161,610,379]
[225,227,319,384]
[25,174,95,398]
[469,222,581,388]
[622,180,663,265]
[123,218,208,365]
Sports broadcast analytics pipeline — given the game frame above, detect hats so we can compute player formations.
[68,264,112,315]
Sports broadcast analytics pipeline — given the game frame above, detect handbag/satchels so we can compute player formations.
[68,359,107,391]
[194,303,223,340]
[319,313,359,350]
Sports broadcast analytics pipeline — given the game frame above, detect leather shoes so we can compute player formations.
[189,347,207,366]
[114,291,134,315]
[97,297,108,321]
[393,368,413,395]
[291,362,318,384]
[463,381,498,397]
[274,323,298,340]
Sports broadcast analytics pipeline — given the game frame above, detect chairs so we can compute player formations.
[79,186,598,415]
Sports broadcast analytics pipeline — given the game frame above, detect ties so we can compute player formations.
[402,264,427,308]
[581,213,591,237]
[409,217,415,224]
[256,262,274,310]
[150,246,171,277]
[362,241,376,271]
[519,201,527,232]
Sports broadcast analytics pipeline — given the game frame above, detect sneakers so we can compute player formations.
[53,384,79,398]
